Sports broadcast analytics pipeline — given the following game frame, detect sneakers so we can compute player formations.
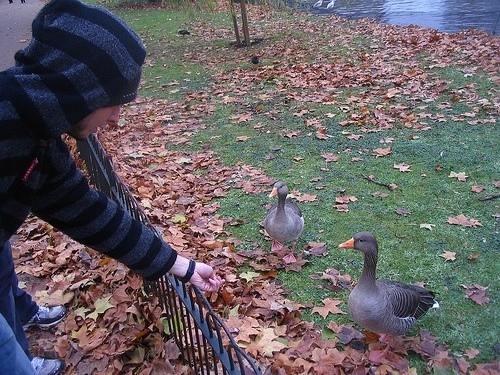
[23,305,66,332]
[30,357,63,375]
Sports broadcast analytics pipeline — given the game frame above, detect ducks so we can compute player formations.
[264,181,304,263]
[337,231,440,364]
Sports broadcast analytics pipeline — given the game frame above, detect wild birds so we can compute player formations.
[313,0,323,8]
[252,55,260,70]
[177,29,191,38]
[326,0,336,10]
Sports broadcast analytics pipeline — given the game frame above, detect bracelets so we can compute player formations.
[180,258,195,283]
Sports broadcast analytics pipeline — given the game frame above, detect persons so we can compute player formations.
[8,0,13,4]
[0,0,225,375]
[21,0,25,3]
[0,315,38,375]
